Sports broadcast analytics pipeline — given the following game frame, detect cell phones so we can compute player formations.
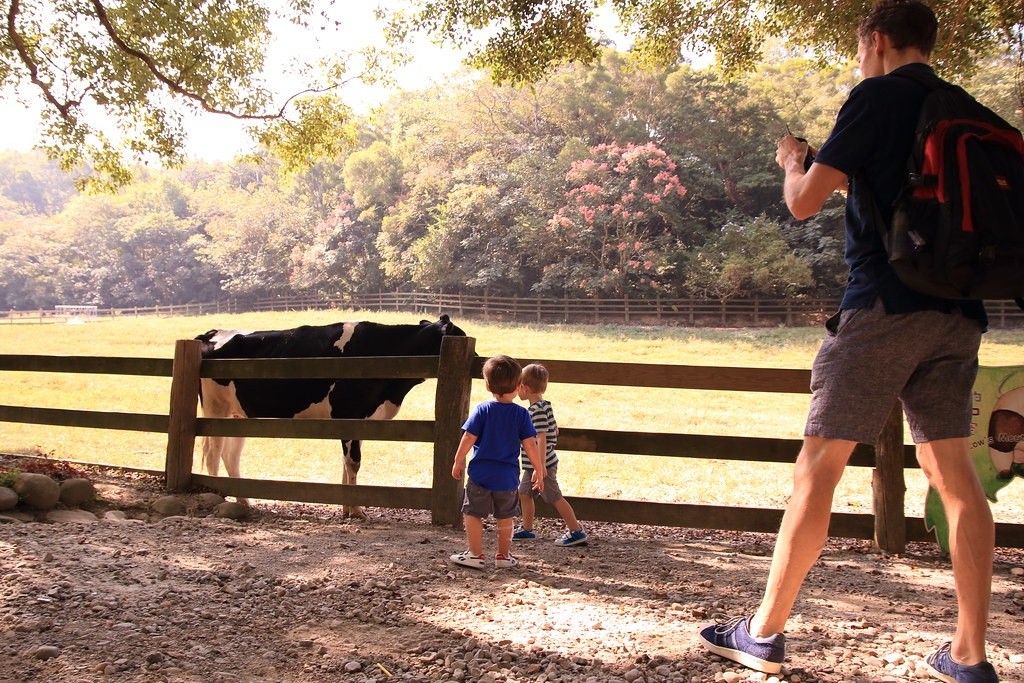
[795,137,814,172]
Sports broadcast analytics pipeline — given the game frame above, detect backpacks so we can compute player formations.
[860,73,1024,300]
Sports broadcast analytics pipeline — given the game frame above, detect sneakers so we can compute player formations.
[698,614,786,675]
[512,525,536,540]
[450,551,486,569]
[495,553,519,567]
[921,640,1000,683]
[554,522,588,546]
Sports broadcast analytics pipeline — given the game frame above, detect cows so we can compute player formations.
[181,314,478,519]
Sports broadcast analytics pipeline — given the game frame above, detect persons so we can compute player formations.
[451,356,544,568]
[511,364,587,546]
[701,0,1003,683]
